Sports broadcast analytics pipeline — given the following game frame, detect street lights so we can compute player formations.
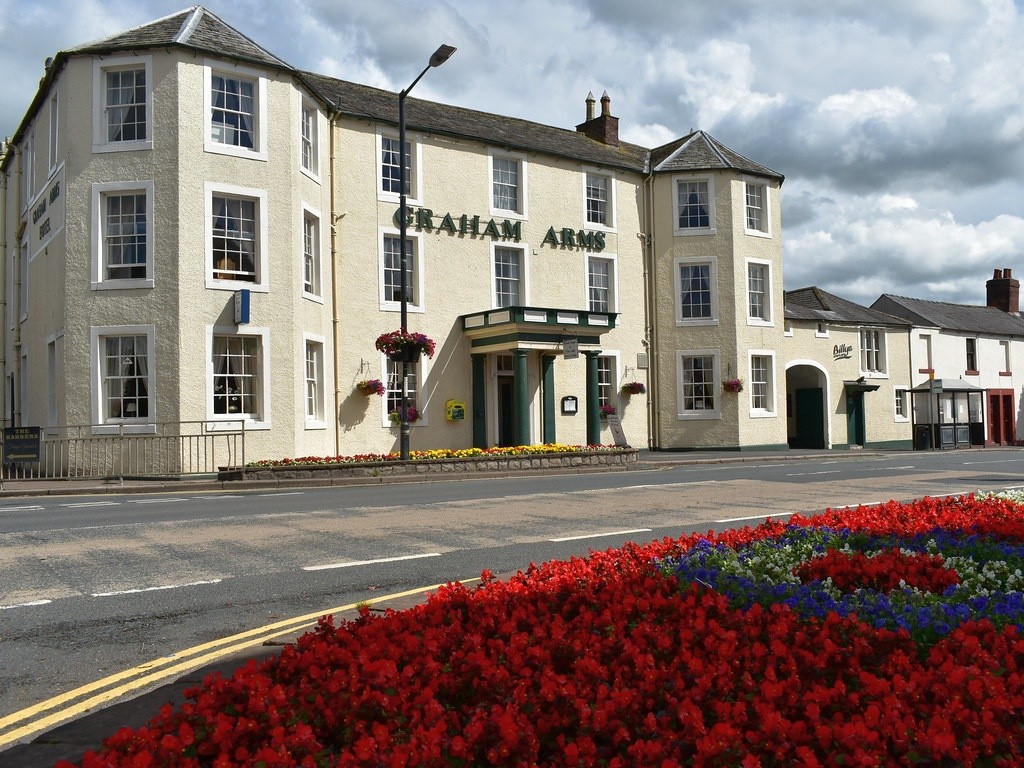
[399,43,458,460]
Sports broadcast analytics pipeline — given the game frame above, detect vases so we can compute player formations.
[361,388,371,397]
[390,343,421,363]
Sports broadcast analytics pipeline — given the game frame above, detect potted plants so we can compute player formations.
[622,383,645,395]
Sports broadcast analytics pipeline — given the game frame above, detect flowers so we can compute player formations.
[389,399,419,427]
[357,377,386,397]
[376,326,435,360]
[722,379,744,392]
[599,405,617,421]
